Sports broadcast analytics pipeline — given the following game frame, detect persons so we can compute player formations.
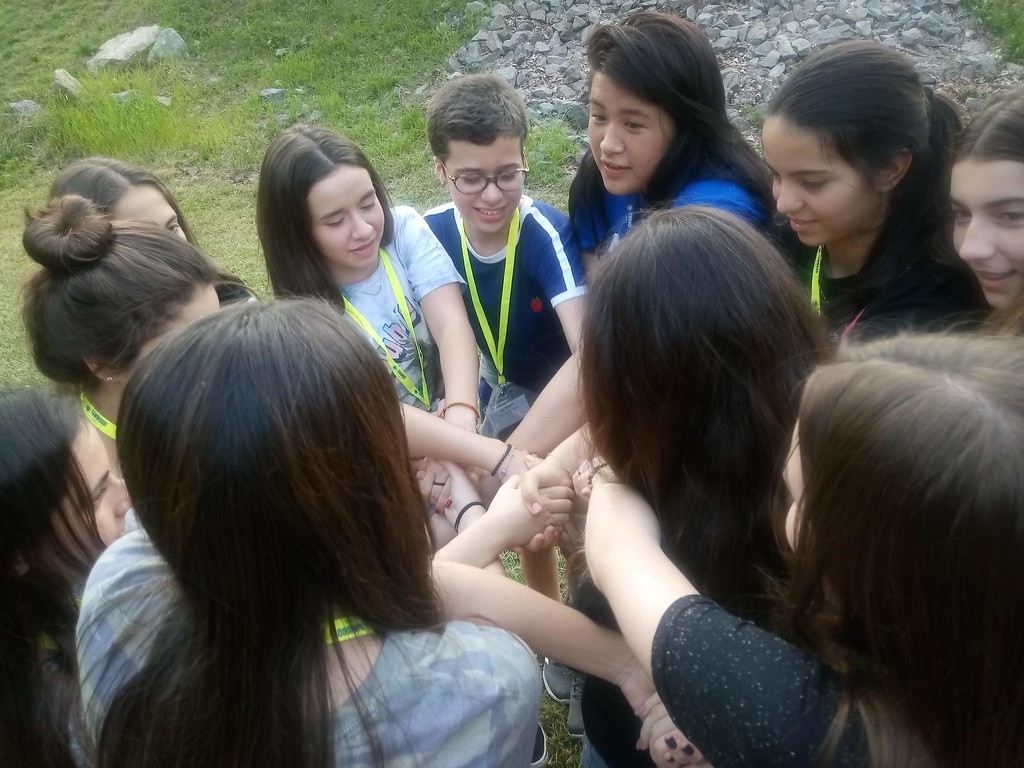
[0,10,1024,768]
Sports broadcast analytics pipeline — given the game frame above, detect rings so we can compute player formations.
[434,482,445,486]
[579,472,581,475]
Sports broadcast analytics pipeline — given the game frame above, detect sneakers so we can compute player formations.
[542,647,572,703]
[566,666,588,739]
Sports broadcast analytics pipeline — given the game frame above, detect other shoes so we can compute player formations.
[530,723,549,768]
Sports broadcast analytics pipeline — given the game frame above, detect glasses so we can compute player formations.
[440,151,530,194]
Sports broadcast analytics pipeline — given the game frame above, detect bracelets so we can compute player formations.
[455,501,486,533]
[588,463,608,484]
[447,402,480,418]
[548,453,571,477]
[491,444,511,476]
[499,448,516,480]
